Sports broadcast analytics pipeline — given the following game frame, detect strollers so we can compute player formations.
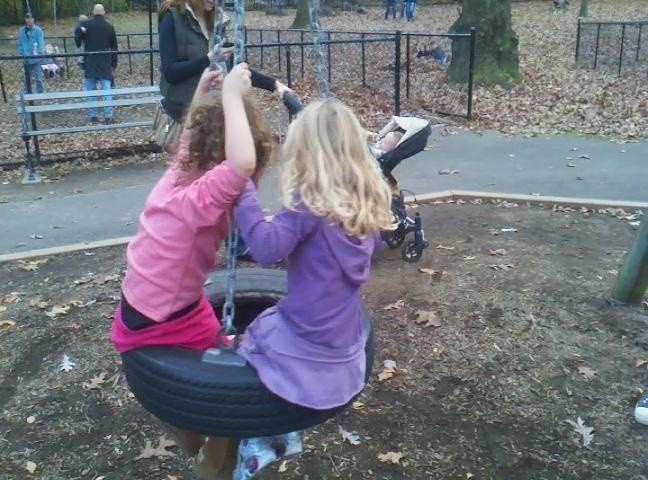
[281,91,432,263]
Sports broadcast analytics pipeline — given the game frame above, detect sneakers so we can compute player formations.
[274,430,303,461]
[91,117,112,125]
[231,437,276,480]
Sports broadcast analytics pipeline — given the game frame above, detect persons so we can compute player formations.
[74,4,118,125]
[110,61,273,480]
[78,14,89,63]
[416,42,430,58]
[429,41,449,63]
[231,96,402,480]
[18,13,45,94]
[384,0,416,22]
[157,0,295,262]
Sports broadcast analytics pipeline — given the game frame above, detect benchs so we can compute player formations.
[15,85,164,181]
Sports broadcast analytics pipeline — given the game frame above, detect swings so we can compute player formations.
[121,0,376,437]
